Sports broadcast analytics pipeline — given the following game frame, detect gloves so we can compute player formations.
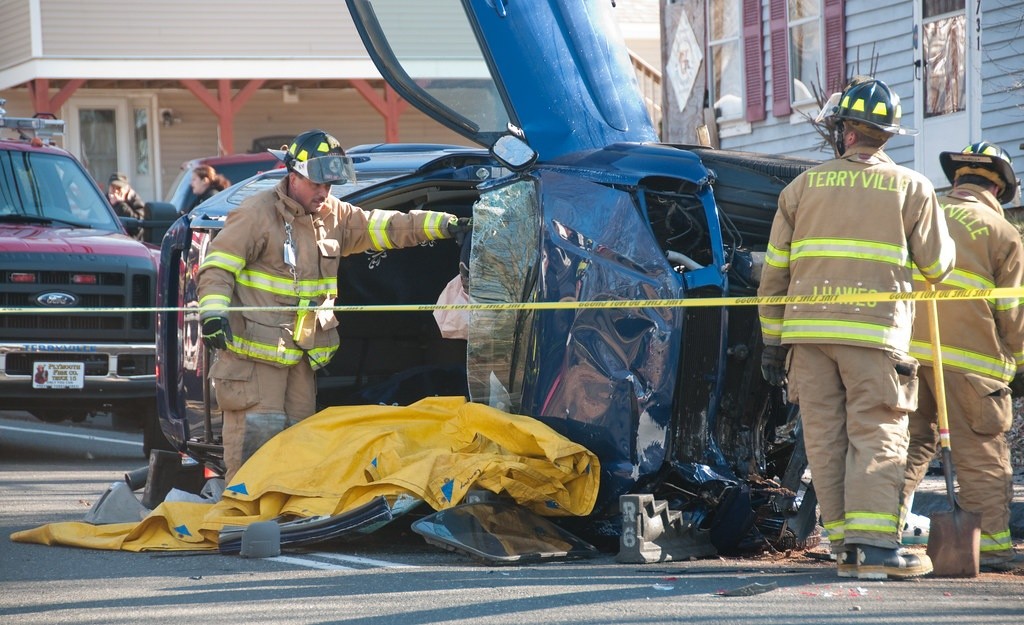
[202,317,233,351]
[448,217,474,247]
[761,346,790,386]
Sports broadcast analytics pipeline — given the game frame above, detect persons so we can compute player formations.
[188,165,230,214]
[757,81,957,578]
[104,172,145,234]
[897,142,1024,570]
[198,129,474,493]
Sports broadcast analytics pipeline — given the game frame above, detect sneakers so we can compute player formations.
[836,552,856,577]
[856,544,935,579]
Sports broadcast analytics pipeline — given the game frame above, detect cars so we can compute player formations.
[151,1,920,554]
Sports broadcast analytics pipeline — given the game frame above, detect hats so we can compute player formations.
[109,172,127,187]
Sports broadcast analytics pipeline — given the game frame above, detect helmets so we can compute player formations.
[939,141,1017,205]
[829,80,919,136]
[268,130,349,186]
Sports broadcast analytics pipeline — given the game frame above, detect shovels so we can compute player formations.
[924,282,982,577]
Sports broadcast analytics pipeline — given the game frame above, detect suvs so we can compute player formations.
[0,100,185,433]
